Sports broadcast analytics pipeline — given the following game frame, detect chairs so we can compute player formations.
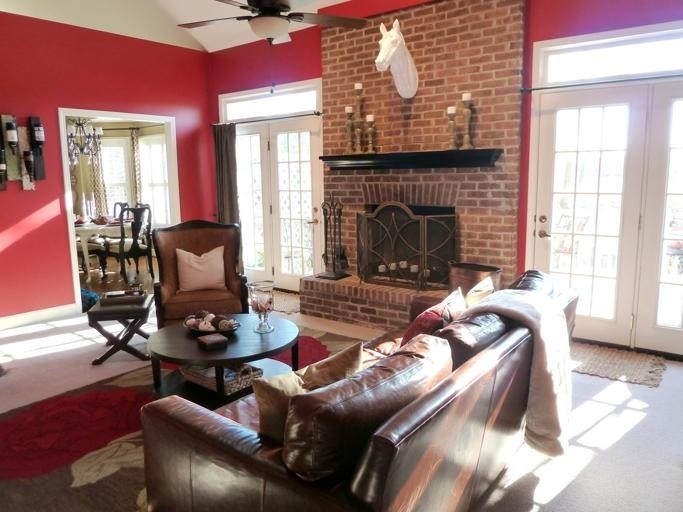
[152,219,250,331]
[74,200,155,286]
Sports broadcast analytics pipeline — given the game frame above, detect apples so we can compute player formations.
[463,93,472,101]
[345,82,375,122]
[447,106,456,114]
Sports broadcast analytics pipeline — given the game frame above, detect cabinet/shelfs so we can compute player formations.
[23,151,35,182]
[0,150,7,179]
[5,122,19,156]
[30,123,46,157]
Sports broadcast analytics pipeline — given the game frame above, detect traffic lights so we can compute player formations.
[175,246,229,293]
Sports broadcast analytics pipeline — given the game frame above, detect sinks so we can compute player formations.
[142,267,583,509]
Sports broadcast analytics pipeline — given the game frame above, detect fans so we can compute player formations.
[176,1,366,45]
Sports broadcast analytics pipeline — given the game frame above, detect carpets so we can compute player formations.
[570,338,667,388]
[0,325,366,512]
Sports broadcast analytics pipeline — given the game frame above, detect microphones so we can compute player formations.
[87,293,155,366]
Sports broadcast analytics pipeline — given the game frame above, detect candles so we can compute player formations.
[345,82,375,122]
[463,93,472,101]
[447,106,456,114]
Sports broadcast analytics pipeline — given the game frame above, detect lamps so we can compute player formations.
[249,16,291,45]
[74,200,155,286]
[152,219,250,331]
[66,117,104,169]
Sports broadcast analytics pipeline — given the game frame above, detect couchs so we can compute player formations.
[142,267,583,509]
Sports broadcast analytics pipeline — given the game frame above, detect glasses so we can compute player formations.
[249,16,291,45]
[66,117,104,169]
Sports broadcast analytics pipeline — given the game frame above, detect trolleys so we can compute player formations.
[176,1,366,45]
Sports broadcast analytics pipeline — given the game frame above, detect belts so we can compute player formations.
[245,280,277,334]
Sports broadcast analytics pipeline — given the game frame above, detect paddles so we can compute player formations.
[0,325,366,512]
[570,338,667,388]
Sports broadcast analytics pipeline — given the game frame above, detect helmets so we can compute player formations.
[182,314,240,335]
[74,218,132,226]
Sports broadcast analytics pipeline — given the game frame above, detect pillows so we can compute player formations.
[465,276,494,306]
[253,340,364,443]
[175,246,229,293]
[399,286,467,349]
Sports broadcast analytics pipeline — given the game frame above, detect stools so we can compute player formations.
[87,293,155,366]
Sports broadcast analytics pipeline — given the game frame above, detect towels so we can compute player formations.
[448,260,503,298]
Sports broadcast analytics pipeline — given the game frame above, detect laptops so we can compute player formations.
[186,310,233,330]
